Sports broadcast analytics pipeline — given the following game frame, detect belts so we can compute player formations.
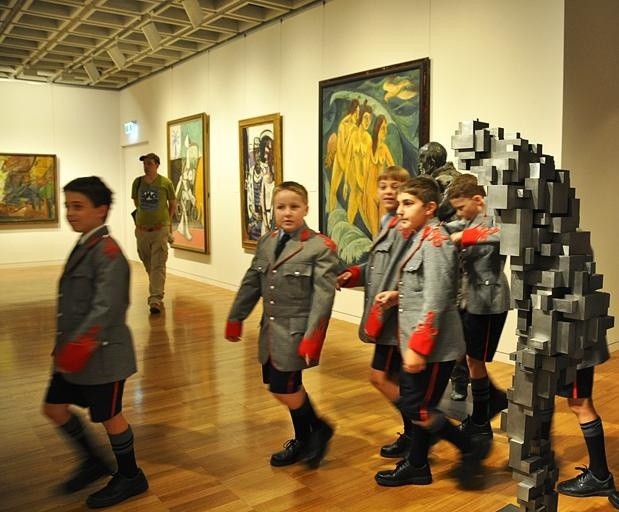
[136,224,163,231]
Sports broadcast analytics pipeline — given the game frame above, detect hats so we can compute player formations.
[139,153,160,163]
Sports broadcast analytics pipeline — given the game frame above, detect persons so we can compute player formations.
[326,96,360,212]
[131,153,175,315]
[336,108,374,236]
[416,141,472,404]
[362,115,397,230]
[335,167,469,458]
[223,181,338,472]
[40,176,148,508]
[443,171,511,430]
[373,176,492,489]
[556,357,615,498]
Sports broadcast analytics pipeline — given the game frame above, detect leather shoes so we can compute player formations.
[557,464,616,497]
[462,389,509,427]
[85,467,148,509]
[455,419,493,443]
[381,431,413,458]
[376,456,432,486]
[270,437,315,467]
[60,453,118,495]
[301,417,335,464]
[608,489,618,509]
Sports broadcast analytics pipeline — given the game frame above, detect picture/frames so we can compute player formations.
[236,112,284,254]
[0,152,59,230]
[316,54,431,295]
[165,112,209,255]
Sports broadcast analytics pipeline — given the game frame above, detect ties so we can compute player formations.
[69,240,82,263]
[275,233,291,259]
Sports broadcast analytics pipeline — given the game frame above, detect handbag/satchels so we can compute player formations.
[131,177,141,225]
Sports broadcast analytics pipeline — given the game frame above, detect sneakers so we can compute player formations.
[150,302,161,313]
[148,294,163,303]
